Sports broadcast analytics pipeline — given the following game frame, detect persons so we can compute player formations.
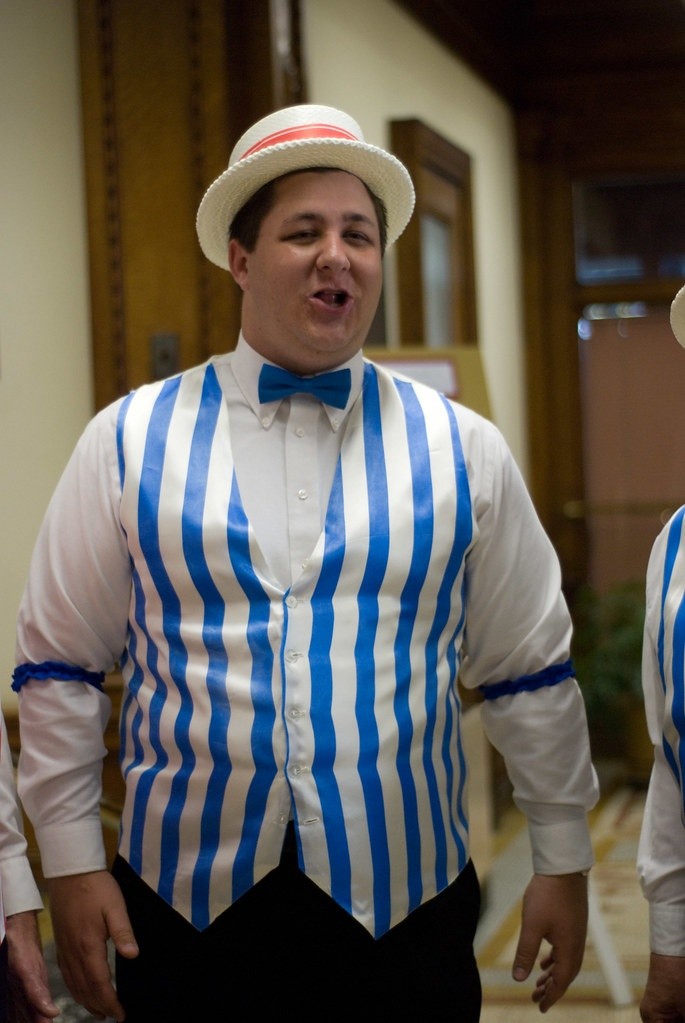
[0,707,60,1023]
[12,104,600,1023]
[635,284,685,1023]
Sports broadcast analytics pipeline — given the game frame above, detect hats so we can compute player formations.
[196,104,415,272]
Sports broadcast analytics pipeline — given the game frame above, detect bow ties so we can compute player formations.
[258,363,353,410]
